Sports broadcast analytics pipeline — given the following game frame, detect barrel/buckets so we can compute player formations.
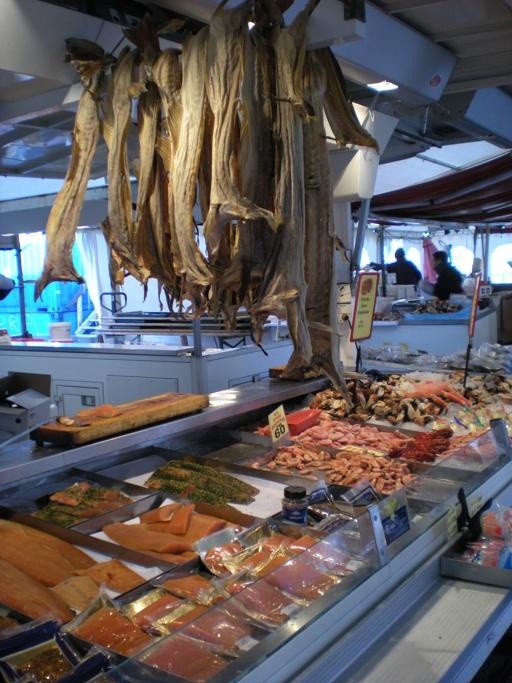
[49,322,71,339]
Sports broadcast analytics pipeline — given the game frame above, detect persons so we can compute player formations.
[371,247,421,290]
[430,251,464,299]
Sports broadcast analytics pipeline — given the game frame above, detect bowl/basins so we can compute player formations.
[284,408,322,435]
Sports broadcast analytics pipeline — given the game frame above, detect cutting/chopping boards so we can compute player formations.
[30,392,211,448]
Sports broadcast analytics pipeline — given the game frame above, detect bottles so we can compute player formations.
[280,484,309,526]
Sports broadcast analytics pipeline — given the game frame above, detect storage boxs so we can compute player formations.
[0,370,52,438]
[373,296,396,314]
[386,283,415,300]
